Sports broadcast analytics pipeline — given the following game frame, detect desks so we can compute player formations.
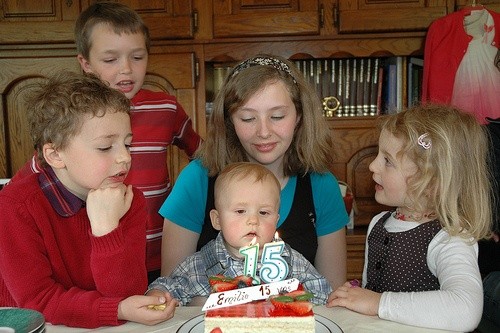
[45,295,462,333]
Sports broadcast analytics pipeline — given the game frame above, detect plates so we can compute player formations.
[0,306,45,333]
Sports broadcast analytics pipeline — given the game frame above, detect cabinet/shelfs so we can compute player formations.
[0,0,499,280]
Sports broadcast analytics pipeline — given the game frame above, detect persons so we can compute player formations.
[143,163,334,305]
[325,104,492,331]
[0,70,179,328]
[74,4,203,284]
[158,53,349,293]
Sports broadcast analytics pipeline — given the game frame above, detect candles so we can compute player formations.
[239,236,261,285]
[259,231,289,283]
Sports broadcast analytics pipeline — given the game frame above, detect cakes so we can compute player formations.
[201,273,315,333]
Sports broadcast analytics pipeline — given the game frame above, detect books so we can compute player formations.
[203,59,424,116]
